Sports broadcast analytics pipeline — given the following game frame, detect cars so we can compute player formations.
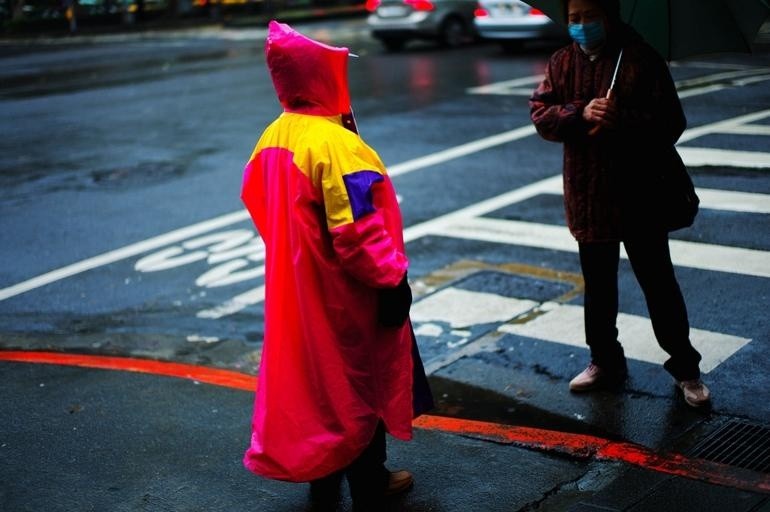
[365,0,474,52]
[473,0,561,51]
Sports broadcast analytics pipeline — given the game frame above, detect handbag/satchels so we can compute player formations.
[590,135,701,234]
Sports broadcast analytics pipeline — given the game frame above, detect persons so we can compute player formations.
[239,20,438,508]
[519,0,713,411]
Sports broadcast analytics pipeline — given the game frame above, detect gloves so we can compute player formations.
[377,270,411,329]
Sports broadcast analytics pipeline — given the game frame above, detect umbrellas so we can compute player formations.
[523,1,770,137]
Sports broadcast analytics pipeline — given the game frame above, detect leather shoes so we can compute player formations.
[569,361,627,394]
[567,22,603,50]
[354,468,412,503]
[676,379,711,406]
